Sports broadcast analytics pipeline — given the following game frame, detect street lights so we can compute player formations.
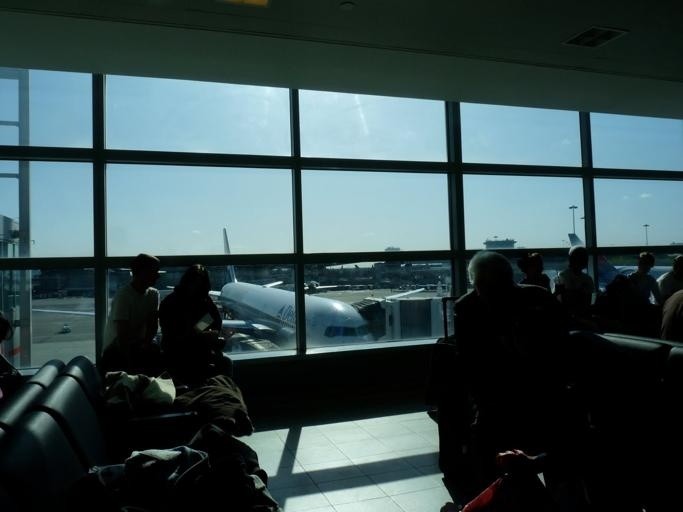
[642,224,651,246]
[568,205,578,233]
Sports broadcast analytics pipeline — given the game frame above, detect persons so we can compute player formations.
[451,245,682,487]
[156,264,233,386]
[95,253,161,381]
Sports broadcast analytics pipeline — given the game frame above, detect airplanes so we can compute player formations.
[31,227,425,350]
[567,234,674,289]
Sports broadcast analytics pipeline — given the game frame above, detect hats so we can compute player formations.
[131,252,160,269]
[631,252,656,267]
[517,251,543,274]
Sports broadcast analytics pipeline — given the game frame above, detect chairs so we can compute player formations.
[0,355,277,511]
[569,328,683,495]
